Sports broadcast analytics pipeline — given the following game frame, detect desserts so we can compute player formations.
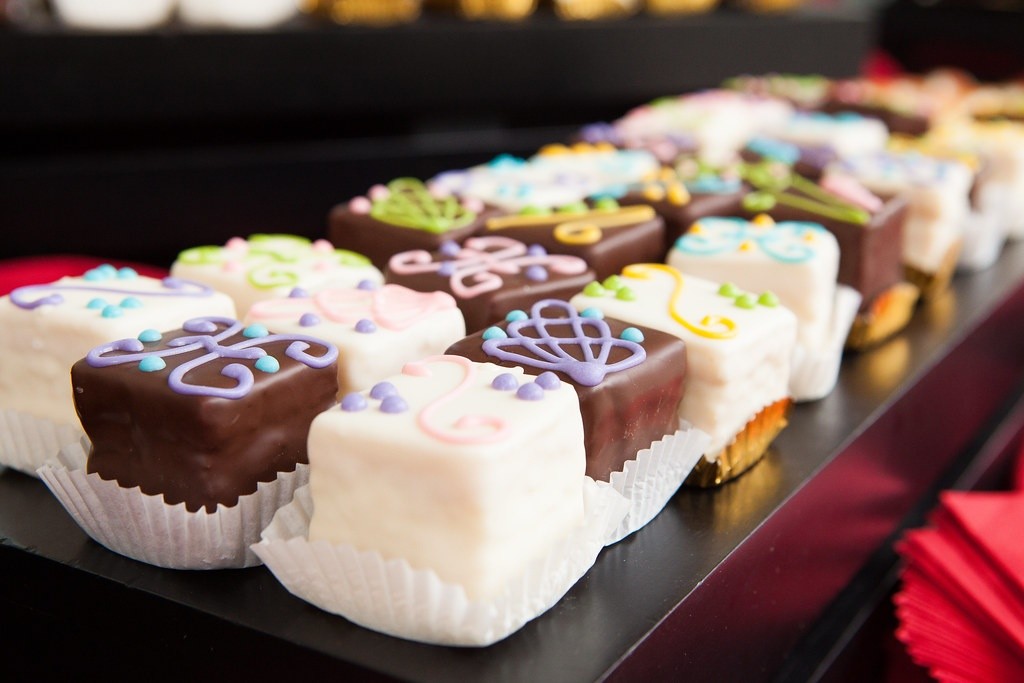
[0,77,1024,649]
